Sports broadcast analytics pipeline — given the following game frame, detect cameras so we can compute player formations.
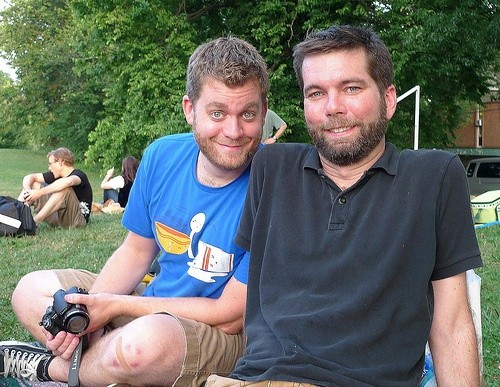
[42,286,91,337]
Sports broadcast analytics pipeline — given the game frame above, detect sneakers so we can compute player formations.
[0,340,53,382]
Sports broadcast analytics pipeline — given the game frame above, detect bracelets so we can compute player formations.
[273,136,277,140]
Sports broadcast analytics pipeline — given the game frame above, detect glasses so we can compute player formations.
[48,162,56,166]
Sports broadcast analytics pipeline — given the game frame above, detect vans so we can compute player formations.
[466,157,500,196]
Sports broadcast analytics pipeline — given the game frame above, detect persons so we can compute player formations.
[0,38,269,387]
[101,156,139,208]
[262,109,287,144]
[18,148,92,228]
[204,24,480,387]
